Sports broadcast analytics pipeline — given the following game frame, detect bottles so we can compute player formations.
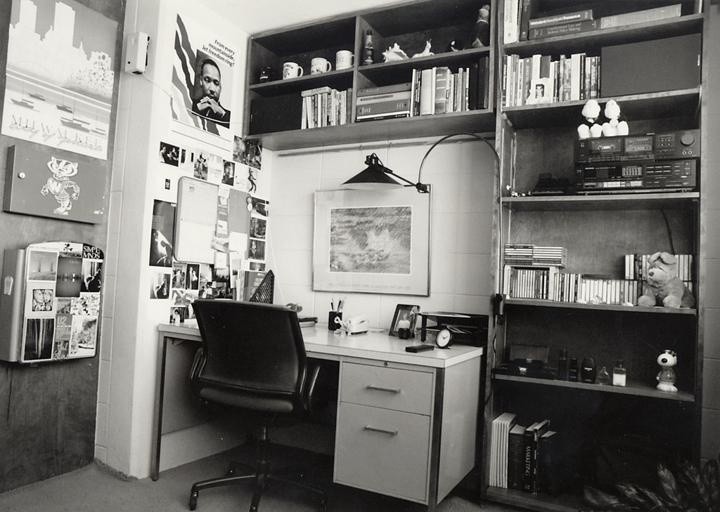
[362,29,375,65]
[597,367,611,386]
[612,360,629,386]
[557,343,568,381]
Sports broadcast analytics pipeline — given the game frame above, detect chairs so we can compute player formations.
[185,298,329,510]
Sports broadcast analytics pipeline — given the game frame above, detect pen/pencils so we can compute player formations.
[330,297,345,312]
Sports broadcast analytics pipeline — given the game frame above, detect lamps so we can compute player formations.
[339,129,501,197]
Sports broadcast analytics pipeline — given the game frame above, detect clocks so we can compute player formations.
[436,328,456,350]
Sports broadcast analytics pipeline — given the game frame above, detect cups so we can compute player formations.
[257,66,275,82]
[335,49,356,70]
[281,63,304,80]
[308,56,332,77]
[328,311,341,330]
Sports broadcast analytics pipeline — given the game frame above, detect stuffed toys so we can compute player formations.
[638,252,697,308]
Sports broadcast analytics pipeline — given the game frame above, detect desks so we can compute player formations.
[150,310,483,512]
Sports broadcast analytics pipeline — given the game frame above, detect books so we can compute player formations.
[355,52,601,124]
[300,86,352,130]
[489,413,551,493]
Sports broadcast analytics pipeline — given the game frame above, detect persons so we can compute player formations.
[395,312,411,331]
[192,59,230,128]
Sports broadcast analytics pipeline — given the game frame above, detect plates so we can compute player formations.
[299,321,316,329]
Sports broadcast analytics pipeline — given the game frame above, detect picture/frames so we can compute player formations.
[389,303,420,338]
[309,185,431,299]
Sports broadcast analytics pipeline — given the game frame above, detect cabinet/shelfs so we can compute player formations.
[239,0,497,154]
[481,1,719,509]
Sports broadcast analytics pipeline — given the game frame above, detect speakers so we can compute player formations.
[124,32,149,77]
[599,32,702,98]
